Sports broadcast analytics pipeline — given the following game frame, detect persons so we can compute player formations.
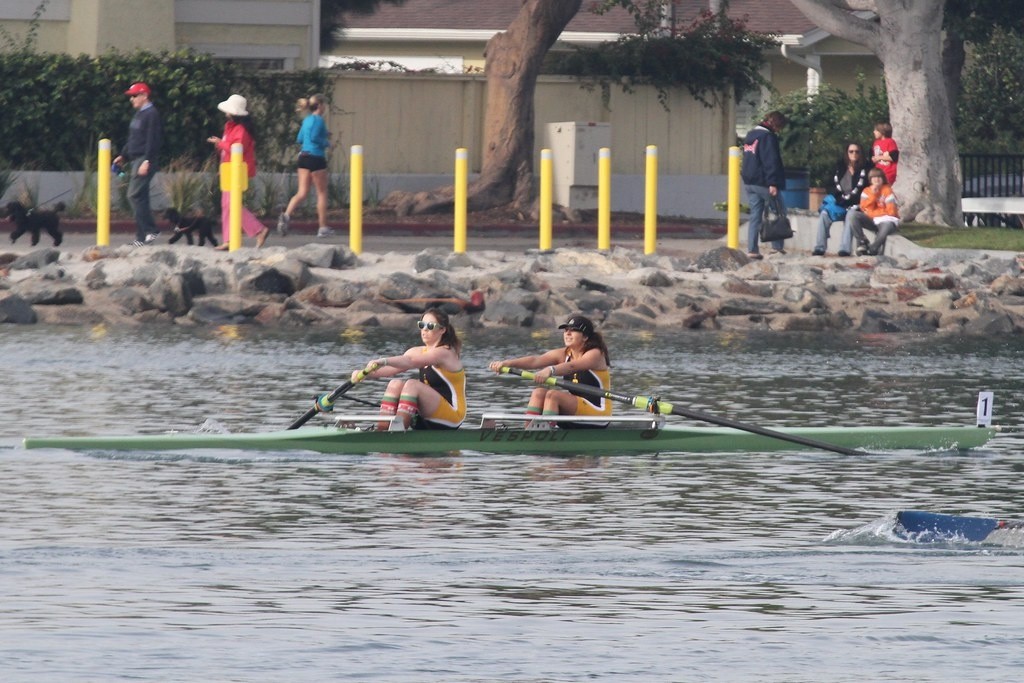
[113,83,170,247]
[871,121,899,187]
[811,141,872,256]
[491,316,612,429]
[741,111,790,260]
[277,94,337,238]
[207,95,269,250]
[351,306,467,429]
[850,168,900,257]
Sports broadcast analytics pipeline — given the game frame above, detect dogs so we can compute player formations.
[161,207,218,247]
[0,200,63,247]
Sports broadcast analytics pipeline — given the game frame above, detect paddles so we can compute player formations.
[488,362,869,456]
[286,362,379,430]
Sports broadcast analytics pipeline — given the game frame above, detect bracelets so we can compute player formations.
[548,366,555,375]
[145,160,151,163]
[379,356,387,366]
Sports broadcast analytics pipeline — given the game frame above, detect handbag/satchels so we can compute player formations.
[760,192,793,242]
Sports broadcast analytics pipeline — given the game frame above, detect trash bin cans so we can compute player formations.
[779,170,810,209]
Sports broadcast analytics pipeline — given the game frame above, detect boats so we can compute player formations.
[895,507,1007,545]
[22,420,1007,457]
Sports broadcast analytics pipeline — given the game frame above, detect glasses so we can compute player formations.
[418,321,443,332]
[847,150,861,154]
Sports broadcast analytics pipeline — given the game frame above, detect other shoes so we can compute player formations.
[839,249,848,257]
[747,251,762,260]
[857,249,874,255]
[771,247,784,253]
[277,212,289,236]
[317,227,334,238]
[145,229,161,243]
[131,239,145,247]
[813,248,823,254]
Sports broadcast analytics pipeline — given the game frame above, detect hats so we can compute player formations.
[217,94,249,116]
[125,82,151,96]
[557,316,592,340]
